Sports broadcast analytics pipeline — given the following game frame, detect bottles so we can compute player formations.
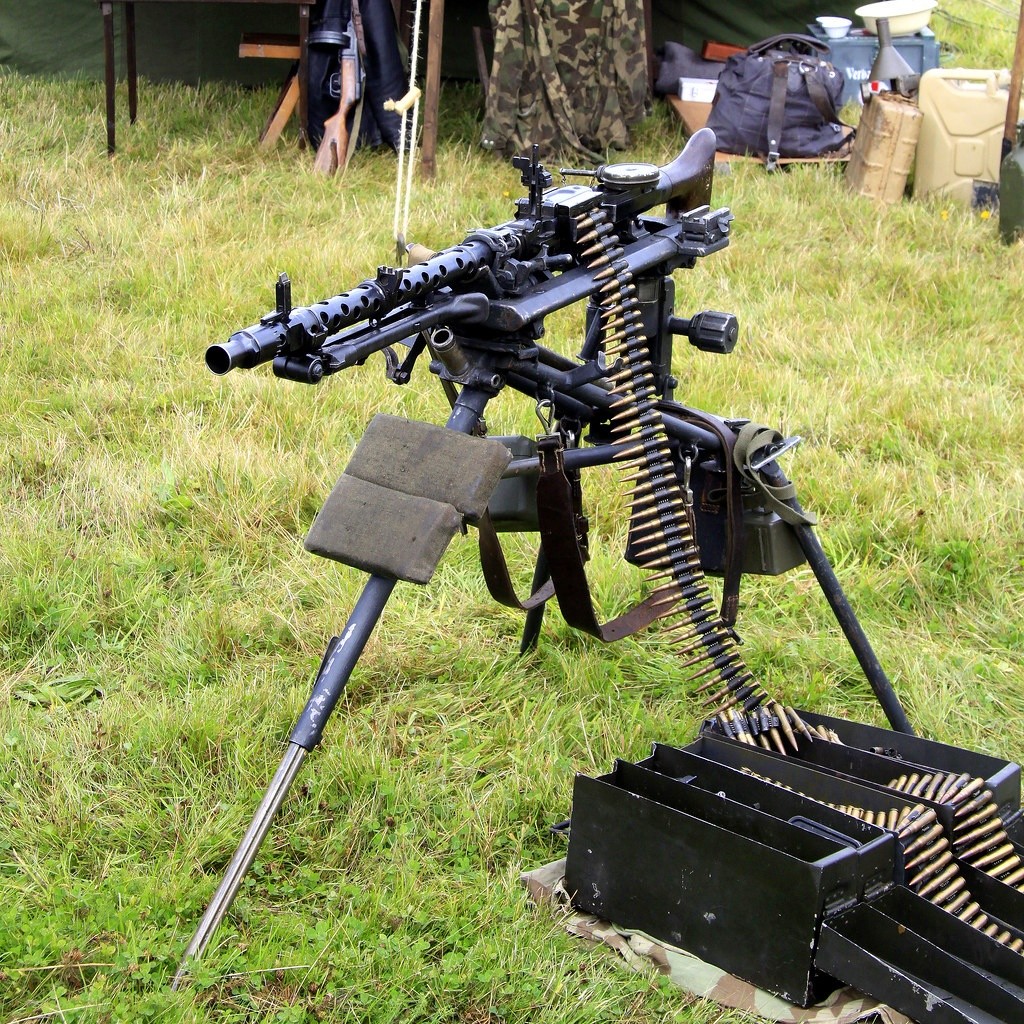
[865,16,917,95]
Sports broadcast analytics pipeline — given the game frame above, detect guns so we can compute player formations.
[308,0,366,177]
[152,119,914,990]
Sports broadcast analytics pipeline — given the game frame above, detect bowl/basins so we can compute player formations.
[855,0,938,37]
[816,17,852,38]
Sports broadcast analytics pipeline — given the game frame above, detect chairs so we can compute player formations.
[239,30,306,151]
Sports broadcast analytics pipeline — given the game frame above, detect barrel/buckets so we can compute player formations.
[844,93,923,205]
[914,68,1023,210]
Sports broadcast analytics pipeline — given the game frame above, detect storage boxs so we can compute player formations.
[668,24,941,207]
